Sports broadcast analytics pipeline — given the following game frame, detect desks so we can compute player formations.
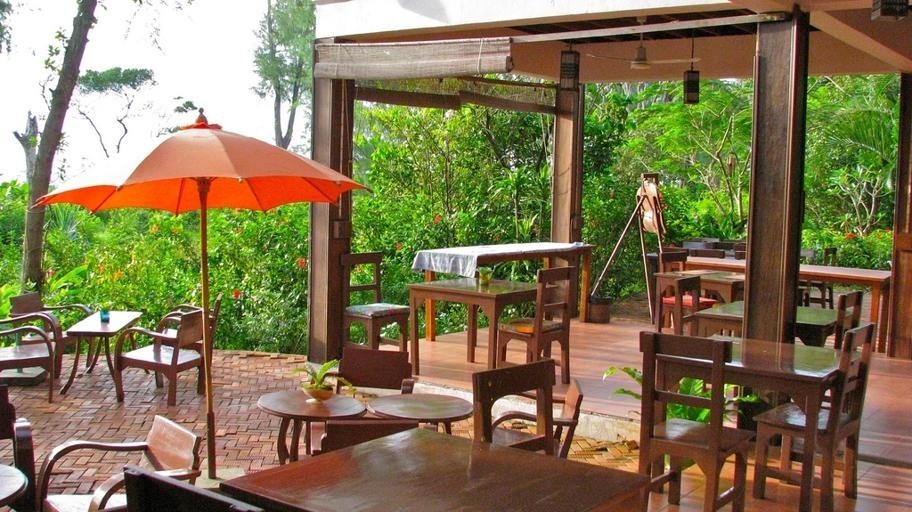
[256,390,366,464]
[59,311,149,396]
[406,278,559,376]
[416,241,597,343]
[1,462,30,512]
[367,393,474,435]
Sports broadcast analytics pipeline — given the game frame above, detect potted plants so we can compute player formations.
[286,359,353,403]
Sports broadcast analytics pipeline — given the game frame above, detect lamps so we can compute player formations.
[683,34,700,104]
[560,41,580,91]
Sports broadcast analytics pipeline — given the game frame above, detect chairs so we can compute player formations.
[0,312,57,402]
[473,359,554,456]
[1,384,36,494]
[121,464,265,511]
[491,382,583,458]
[114,291,224,405]
[220,426,649,512]
[497,264,578,384]
[9,291,93,377]
[341,251,410,354]
[309,418,418,452]
[36,415,202,512]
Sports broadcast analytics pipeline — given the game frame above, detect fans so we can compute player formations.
[581,17,701,70]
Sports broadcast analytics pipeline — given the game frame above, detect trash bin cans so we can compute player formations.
[588,296,612,323]
